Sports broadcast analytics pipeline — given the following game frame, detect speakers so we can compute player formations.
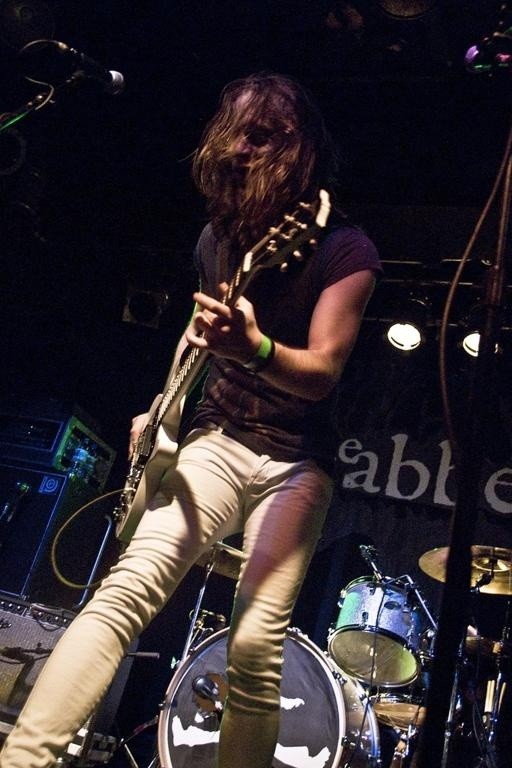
[0,466,110,603]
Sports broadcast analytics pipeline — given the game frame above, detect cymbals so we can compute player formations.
[194,540,243,579]
[418,545,512,595]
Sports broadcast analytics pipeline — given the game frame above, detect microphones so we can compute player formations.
[1,646,21,655]
[465,28,505,72]
[59,41,123,94]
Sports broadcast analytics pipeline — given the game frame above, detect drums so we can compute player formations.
[327,574,434,688]
[156,627,383,768]
[365,661,434,739]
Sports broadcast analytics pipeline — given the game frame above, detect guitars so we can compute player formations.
[113,189,331,543]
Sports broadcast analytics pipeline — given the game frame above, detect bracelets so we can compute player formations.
[245,339,276,374]
[242,333,272,371]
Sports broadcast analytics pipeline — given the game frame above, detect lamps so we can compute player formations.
[382,262,502,363]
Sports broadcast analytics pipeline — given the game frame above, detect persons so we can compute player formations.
[0,71,384,768]
